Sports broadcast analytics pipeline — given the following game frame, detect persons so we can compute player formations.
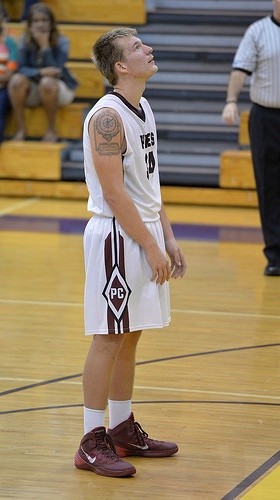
[0,4,19,144]
[8,3,79,143]
[221,0,280,276]
[74,26,187,477]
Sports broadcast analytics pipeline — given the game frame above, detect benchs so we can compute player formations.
[0,0,274,189]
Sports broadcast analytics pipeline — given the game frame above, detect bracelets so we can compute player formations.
[226,99,238,103]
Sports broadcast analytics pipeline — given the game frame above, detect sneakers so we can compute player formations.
[74,426,136,477]
[264,262,280,276]
[107,411,178,458]
